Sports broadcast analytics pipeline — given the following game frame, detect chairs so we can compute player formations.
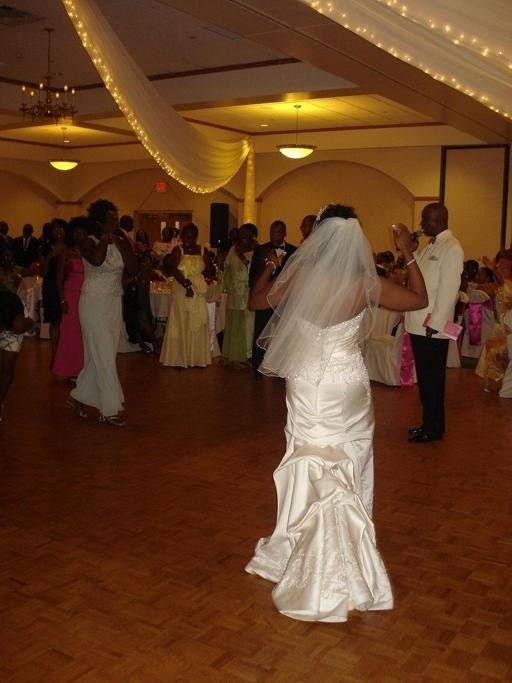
[365,322,419,386]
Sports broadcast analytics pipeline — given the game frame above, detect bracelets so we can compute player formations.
[403,257,415,266]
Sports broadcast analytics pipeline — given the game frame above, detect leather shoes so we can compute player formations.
[409,433,441,442]
[408,424,426,433]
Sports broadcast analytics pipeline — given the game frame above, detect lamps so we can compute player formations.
[18,75,78,125]
[48,157,79,171]
[276,144,317,159]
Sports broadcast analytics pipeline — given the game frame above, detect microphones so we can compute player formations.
[396,231,422,251]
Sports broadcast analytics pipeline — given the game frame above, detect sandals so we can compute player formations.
[67,395,88,420]
[96,415,126,426]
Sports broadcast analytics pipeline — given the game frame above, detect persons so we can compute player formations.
[159,224,212,370]
[455,258,478,314]
[246,202,430,623]
[114,214,139,342]
[222,223,261,370]
[152,228,174,257]
[13,224,40,267]
[476,249,511,395]
[373,251,393,277]
[42,217,69,369]
[1,221,17,264]
[300,215,317,242]
[37,222,52,253]
[477,267,496,319]
[1,257,36,404]
[71,199,125,425]
[396,203,465,442]
[53,215,87,382]
[249,221,299,379]
